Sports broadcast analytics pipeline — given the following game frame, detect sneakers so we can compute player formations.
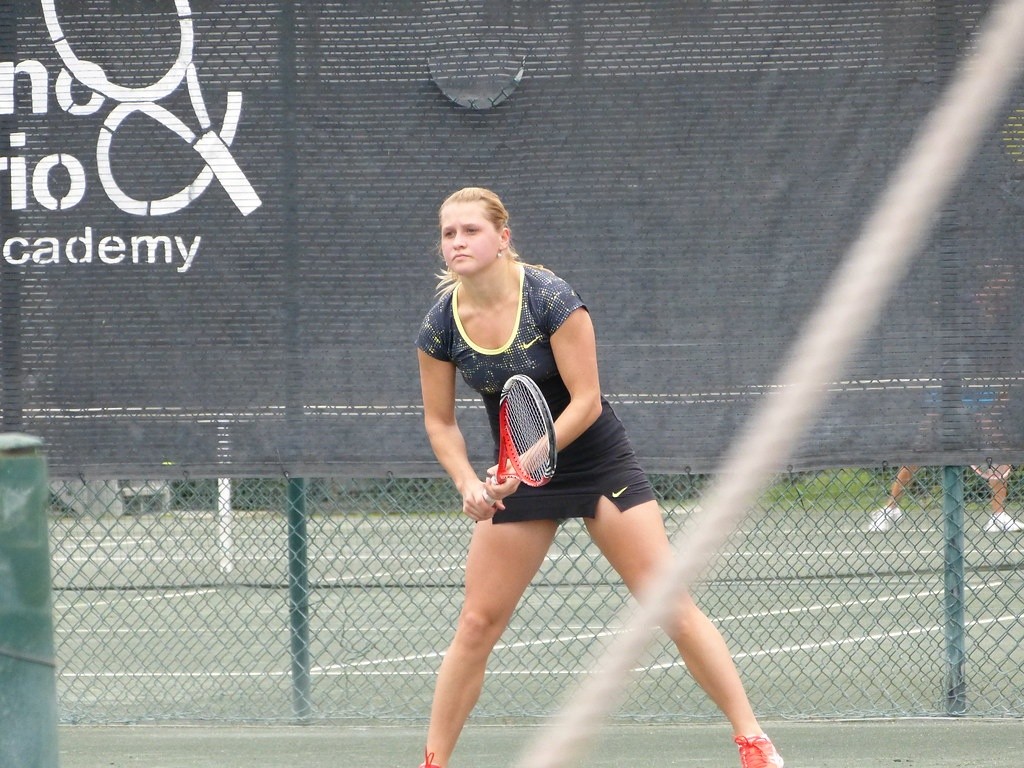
[983,512,1024,532]
[869,505,902,532]
[735,734,784,768]
[416,747,447,768]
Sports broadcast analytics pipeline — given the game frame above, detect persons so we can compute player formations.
[411,186,785,767]
[866,258,1024,537]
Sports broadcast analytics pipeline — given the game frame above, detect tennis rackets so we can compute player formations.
[482,374,559,508]
